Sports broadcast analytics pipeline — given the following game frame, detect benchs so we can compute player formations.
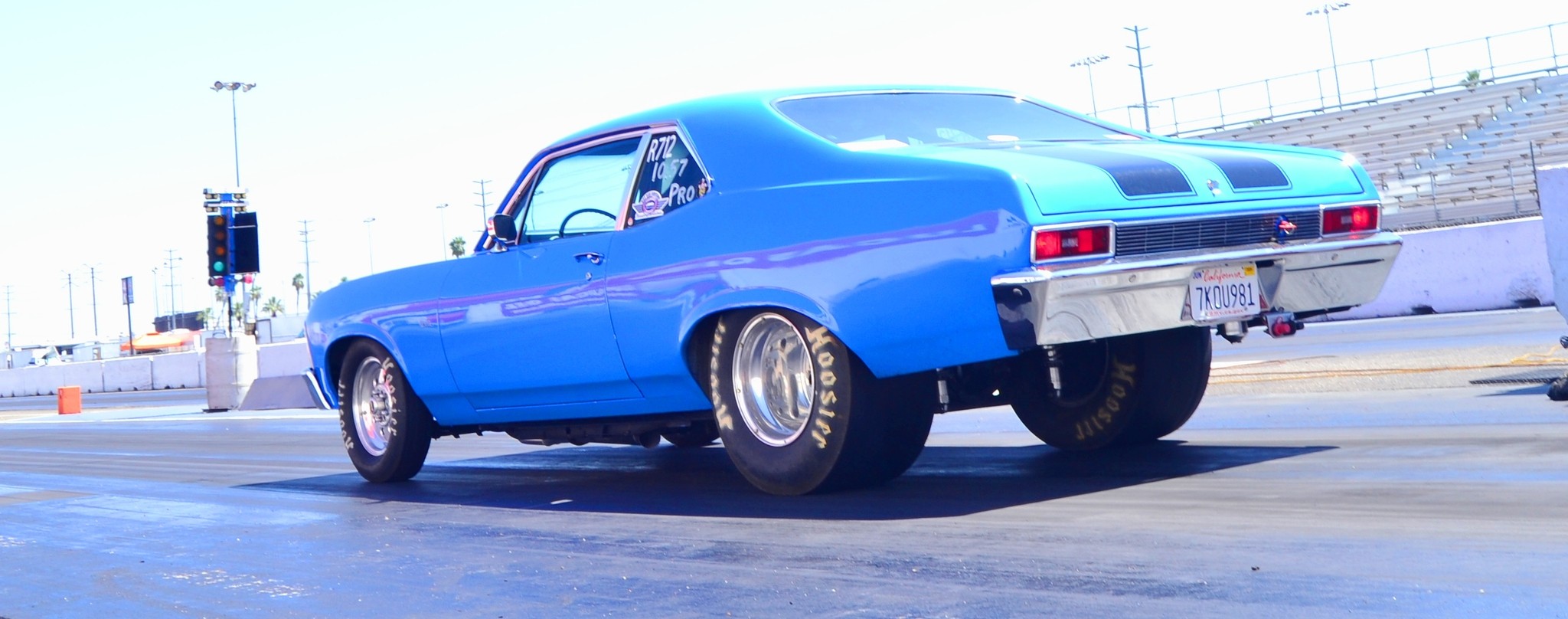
[1183,73,1568,208]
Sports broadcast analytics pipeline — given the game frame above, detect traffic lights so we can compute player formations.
[208,214,226,276]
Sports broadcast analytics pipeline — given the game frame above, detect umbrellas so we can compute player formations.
[121,332,185,350]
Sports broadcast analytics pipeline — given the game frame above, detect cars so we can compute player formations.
[302,84,1405,498]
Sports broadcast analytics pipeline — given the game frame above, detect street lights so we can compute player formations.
[1071,53,1110,118]
[152,249,182,328]
[211,80,257,328]
[1306,2,1351,110]
